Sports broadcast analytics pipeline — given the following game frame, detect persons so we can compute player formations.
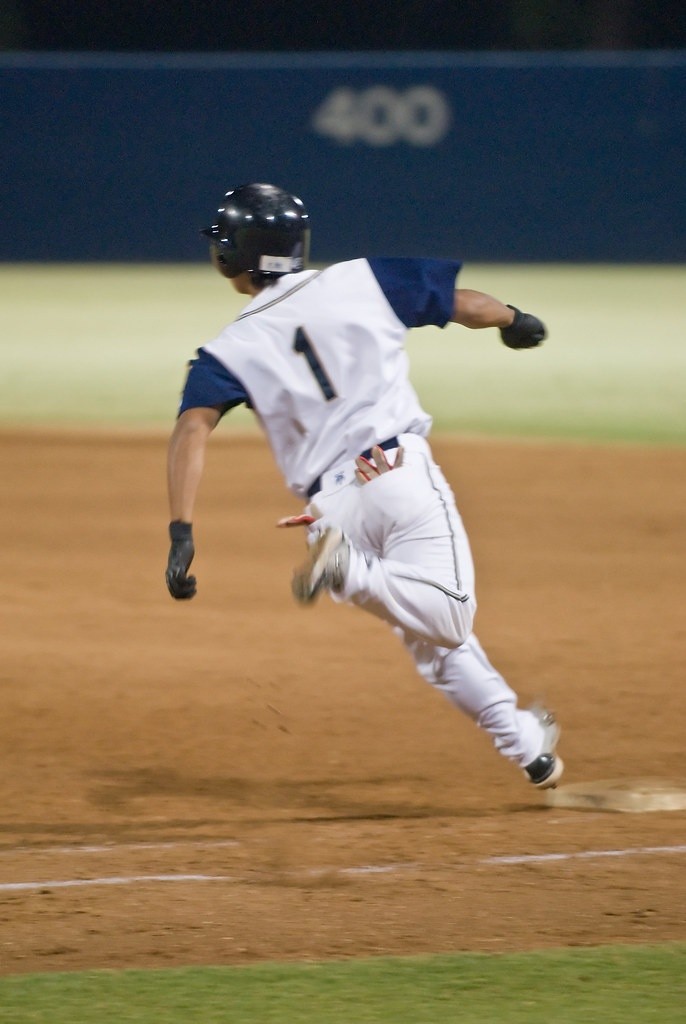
[165,183,565,792]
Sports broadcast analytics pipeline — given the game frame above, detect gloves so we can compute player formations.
[165,520,196,600]
[499,304,546,349]
[354,446,404,485]
[276,514,316,528]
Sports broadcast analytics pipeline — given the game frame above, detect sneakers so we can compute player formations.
[292,526,349,605]
[525,703,563,790]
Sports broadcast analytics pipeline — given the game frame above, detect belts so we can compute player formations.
[306,436,398,496]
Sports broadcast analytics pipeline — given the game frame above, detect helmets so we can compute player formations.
[199,182,309,284]
